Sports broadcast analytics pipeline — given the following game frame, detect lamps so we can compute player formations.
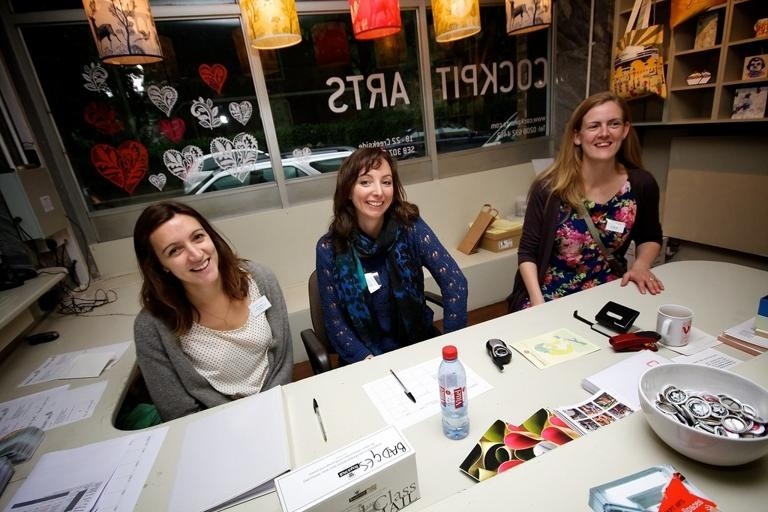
[80,0,164,66]
[348,0,402,41]
[504,0,551,37]
[237,0,302,50]
[430,0,482,43]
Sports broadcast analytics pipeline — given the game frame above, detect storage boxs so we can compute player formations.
[468,216,524,254]
[273,419,420,512]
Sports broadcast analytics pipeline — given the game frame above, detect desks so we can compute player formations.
[0,260,767,512]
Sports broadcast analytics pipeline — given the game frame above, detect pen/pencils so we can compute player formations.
[389,369,417,404]
[313,398,327,442]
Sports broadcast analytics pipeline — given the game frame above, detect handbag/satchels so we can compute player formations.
[610,24,668,102]
[456,203,498,255]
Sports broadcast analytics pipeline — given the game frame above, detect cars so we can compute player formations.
[372,119,503,162]
[184,149,273,195]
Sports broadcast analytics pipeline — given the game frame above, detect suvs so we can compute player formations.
[183,145,360,197]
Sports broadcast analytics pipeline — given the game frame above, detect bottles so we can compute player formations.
[437,346,472,445]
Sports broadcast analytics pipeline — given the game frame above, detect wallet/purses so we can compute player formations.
[595,302,639,334]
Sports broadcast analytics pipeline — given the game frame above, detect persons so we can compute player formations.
[503,92,662,315]
[131,200,294,423]
[314,144,469,366]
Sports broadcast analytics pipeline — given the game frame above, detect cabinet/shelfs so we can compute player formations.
[611,0,767,130]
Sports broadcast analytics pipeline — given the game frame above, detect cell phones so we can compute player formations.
[486,339,512,370]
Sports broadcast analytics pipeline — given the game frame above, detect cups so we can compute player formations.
[655,305,693,349]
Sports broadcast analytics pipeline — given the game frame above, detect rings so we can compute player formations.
[649,275,653,281]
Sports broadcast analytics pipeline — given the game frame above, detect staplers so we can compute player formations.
[610,332,662,353]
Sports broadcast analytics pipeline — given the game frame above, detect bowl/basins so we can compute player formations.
[639,361,767,469]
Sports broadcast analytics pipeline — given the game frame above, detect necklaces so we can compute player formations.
[187,291,232,329]
